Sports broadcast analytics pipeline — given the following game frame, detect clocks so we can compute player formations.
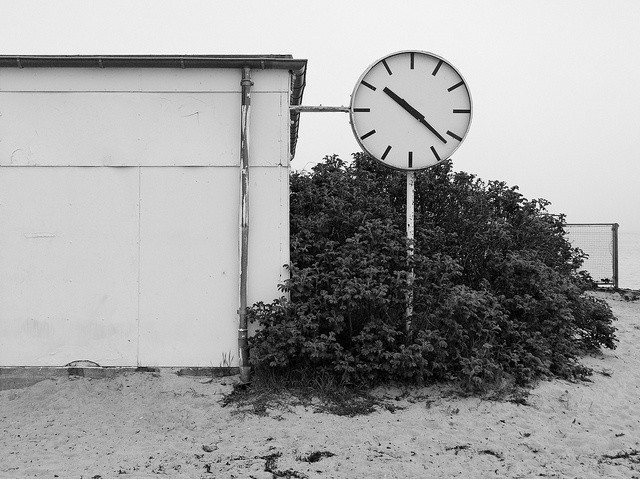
[347,50,473,172]
